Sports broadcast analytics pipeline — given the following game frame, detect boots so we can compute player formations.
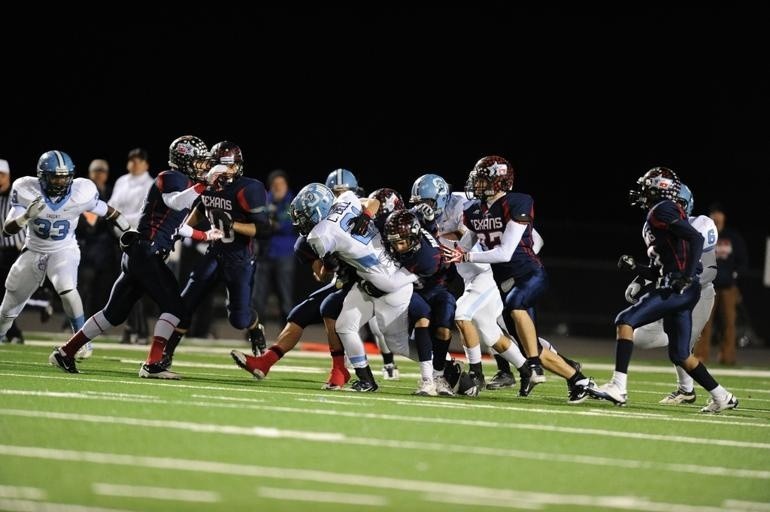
[321,368,352,391]
[230,349,271,380]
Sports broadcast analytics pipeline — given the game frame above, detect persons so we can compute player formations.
[1,143,137,364]
[159,137,599,408]
[619,183,720,407]
[50,132,239,386]
[61,157,118,329]
[694,207,738,367]
[583,165,739,415]
[103,146,156,348]
[1,157,54,347]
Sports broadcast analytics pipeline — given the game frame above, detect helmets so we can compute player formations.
[168,135,212,182]
[464,155,515,201]
[628,167,682,208]
[408,173,451,217]
[367,188,405,215]
[289,183,338,239]
[382,208,423,258]
[210,140,244,187]
[36,150,76,197]
[675,183,695,217]
[325,168,359,193]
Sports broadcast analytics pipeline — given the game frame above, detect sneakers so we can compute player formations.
[76,341,94,359]
[48,346,79,374]
[247,323,268,357]
[2,326,24,344]
[159,351,174,368]
[414,377,435,396]
[567,377,598,404]
[658,387,697,405]
[516,358,546,398]
[700,391,739,413]
[433,362,484,398]
[138,362,180,380]
[383,365,398,380]
[587,381,628,407]
[485,369,516,390]
[39,294,217,345]
[341,378,379,392]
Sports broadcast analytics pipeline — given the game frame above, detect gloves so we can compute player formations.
[616,254,636,274]
[669,275,693,295]
[206,164,228,185]
[211,208,234,230]
[322,250,341,272]
[15,195,47,229]
[432,241,468,266]
[203,227,224,242]
[624,275,645,304]
[346,212,373,236]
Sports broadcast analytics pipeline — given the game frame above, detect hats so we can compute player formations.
[88,159,109,172]
[128,148,148,161]
[0,158,10,175]
